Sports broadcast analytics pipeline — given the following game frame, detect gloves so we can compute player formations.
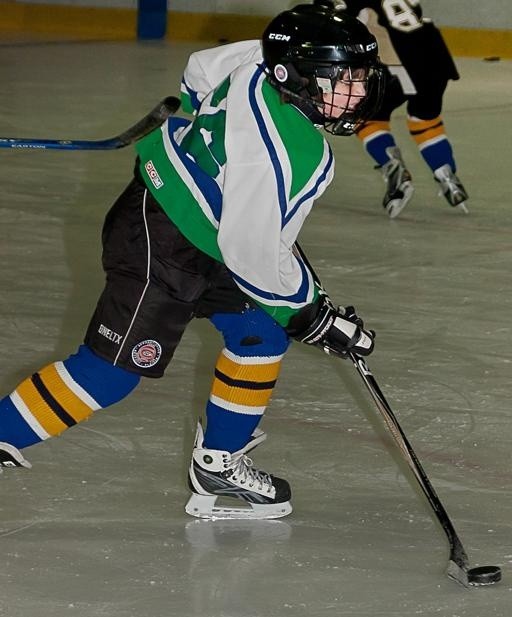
[294,300,376,359]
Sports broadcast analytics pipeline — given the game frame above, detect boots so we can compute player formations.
[188,418,292,504]
[373,146,414,215]
[433,164,469,207]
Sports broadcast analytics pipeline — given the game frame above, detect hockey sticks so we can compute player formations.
[291,239,502,587]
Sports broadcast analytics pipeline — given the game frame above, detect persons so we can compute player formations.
[312,0,471,215]
[0,1,385,505]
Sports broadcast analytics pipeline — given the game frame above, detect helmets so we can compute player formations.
[262,4,379,96]
